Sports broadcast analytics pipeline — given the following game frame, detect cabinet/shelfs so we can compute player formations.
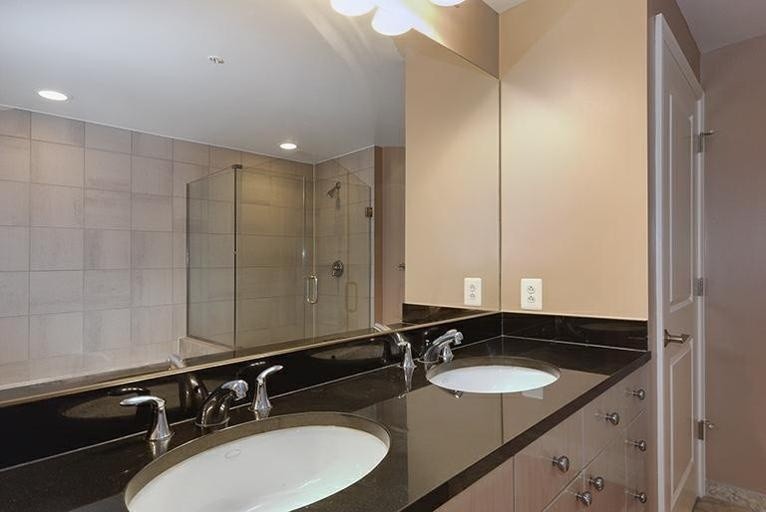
[434,361,657,512]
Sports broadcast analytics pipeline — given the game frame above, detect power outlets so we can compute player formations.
[521,278,543,310]
[463,277,482,308]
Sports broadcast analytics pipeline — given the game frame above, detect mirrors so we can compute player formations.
[0,0,502,403]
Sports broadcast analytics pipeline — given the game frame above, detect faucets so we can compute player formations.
[179,372,209,414]
[390,333,407,360]
[167,355,186,370]
[418,331,463,364]
[195,379,249,428]
[370,322,390,332]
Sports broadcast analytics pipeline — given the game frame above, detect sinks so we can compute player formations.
[311,346,400,360]
[124,411,390,512]
[425,355,562,395]
[62,380,226,419]
